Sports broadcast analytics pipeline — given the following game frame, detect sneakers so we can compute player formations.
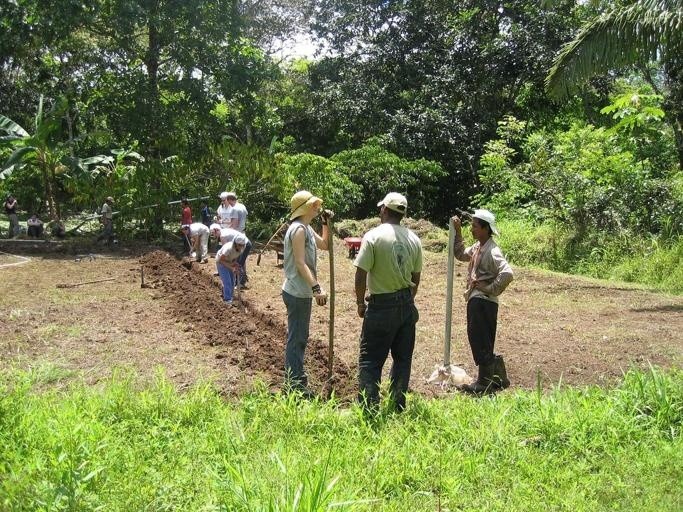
[234,284,245,290]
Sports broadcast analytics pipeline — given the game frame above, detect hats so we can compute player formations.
[472,209,499,236]
[233,234,245,245]
[210,223,222,230]
[290,190,323,221]
[376,192,408,214]
[219,191,235,198]
[106,196,115,203]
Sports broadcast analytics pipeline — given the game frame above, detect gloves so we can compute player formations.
[320,209,335,225]
[312,283,328,306]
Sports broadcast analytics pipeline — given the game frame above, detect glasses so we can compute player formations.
[220,200,226,201]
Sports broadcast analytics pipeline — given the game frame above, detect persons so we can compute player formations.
[281,190,336,393]
[6,195,21,238]
[312,284,321,294]
[451,210,513,393]
[50,214,65,237]
[180,191,253,306]
[27,214,44,239]
[354,191,423,413]
[102,196,114,238]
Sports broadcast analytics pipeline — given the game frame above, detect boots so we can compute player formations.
[462,362,495,394]
[493,355,510,392]
